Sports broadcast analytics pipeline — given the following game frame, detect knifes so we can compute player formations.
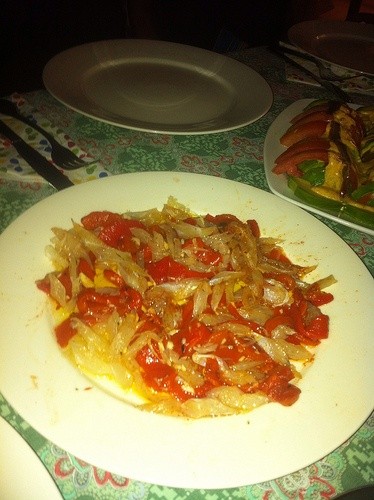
[0,120,73,189]
[267,47,352,104]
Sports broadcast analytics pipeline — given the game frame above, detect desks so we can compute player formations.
[0,46,372,500]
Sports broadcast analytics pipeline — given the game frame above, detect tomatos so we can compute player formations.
[271,101,366,193]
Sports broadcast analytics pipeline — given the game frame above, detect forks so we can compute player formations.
[275,44,365,81]
[1,97,100,172]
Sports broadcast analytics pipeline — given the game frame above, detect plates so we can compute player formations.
[263,95,374,240]
[0,169,374,489]
[288,19,374,78]
[41,42,274,138]
[0,416,64,499]
